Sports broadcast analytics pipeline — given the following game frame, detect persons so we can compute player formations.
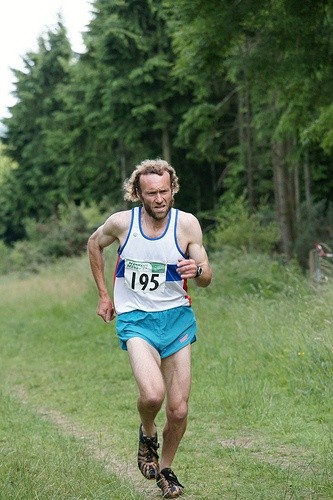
[86,159,213,498]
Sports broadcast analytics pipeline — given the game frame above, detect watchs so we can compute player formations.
[195,266,202,278]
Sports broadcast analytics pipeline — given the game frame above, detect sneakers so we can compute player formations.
[137,420,159,481]
[154,467,184,499]
